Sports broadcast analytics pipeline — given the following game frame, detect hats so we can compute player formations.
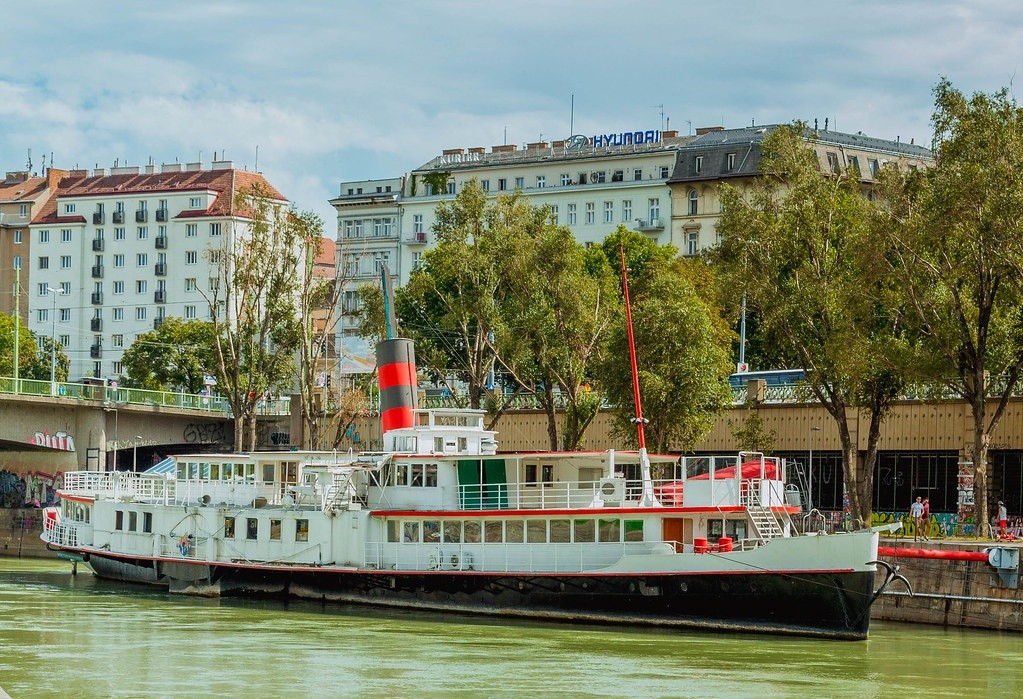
[998,501,1003,504]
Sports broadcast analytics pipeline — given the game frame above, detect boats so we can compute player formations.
[36,237,880,639]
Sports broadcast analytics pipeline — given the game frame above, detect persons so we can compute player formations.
[995,501,1008,538]
[908,496,931,541]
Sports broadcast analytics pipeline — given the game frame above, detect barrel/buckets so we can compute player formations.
[694,538,707,553]
[719,538,732,552]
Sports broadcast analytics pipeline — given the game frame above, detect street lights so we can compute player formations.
[46,286,65,394]
[809,426,821,532]
[322,290,346,450]
[737,238,760,385]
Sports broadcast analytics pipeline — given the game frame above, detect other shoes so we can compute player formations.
[920,538,924,541]
[925,536,928,541]
